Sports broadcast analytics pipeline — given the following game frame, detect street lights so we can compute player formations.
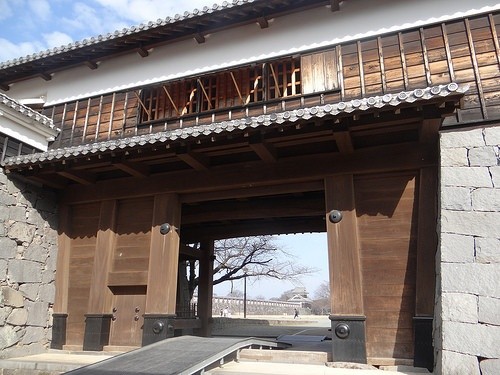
[243,268,248,318]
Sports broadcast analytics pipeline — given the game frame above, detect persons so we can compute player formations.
[294,309,298,320]
[220,308,228,316]
